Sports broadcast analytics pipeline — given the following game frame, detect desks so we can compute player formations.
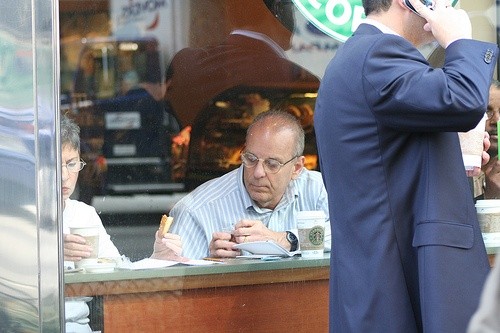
[64,247,498,333]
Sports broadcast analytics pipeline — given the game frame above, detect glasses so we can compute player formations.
[240,146,299,174]
[486,109,500,120]
[62,158,87,172]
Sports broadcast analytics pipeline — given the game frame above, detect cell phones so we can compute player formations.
[403,0,435,19]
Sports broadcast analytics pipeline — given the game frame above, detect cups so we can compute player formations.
[69,225,100,270]
[475,199,500,247]
[457,112,488,171]
[296,210,327,260]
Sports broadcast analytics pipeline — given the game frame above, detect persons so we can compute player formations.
[85,0,323,184]
[168,111,333,261]
[313,1,500,333]
[1,1,187,333]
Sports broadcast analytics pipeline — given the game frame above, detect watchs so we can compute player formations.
[286,231,298,252]
